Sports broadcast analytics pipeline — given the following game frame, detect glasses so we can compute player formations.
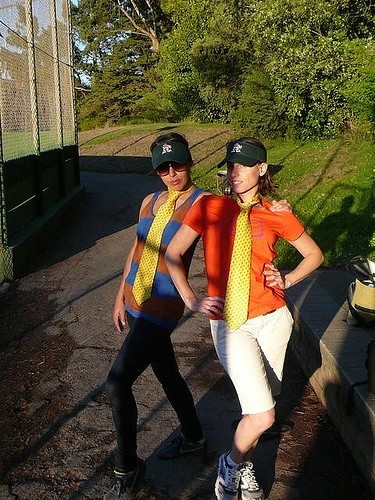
[156,162,189,176]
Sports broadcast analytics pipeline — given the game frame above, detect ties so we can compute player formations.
[223,193,261,332]
[131,183,194,306]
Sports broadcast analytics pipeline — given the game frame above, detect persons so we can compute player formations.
[101,132,293,500]
[163,137,325,500]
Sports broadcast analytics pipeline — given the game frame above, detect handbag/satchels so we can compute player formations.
[346,254,375,326]
[364,339,375,394]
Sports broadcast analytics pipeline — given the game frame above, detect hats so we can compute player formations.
[152,141,192,169]
[217,142,267,168]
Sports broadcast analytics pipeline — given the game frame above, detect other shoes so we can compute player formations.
[263,423,291,434]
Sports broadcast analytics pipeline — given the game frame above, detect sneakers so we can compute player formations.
[103,458,146,500]
[214,449,244,500]
[239,460,265,500]
[157,432,206,459]
